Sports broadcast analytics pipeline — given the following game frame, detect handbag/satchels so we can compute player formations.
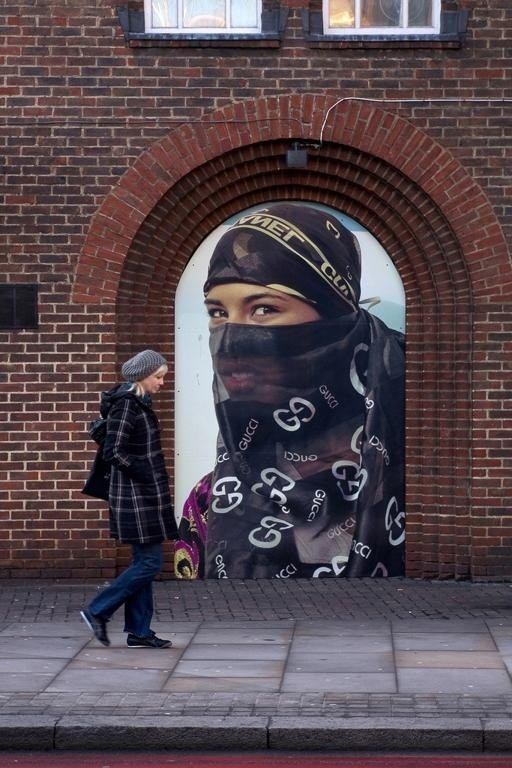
[80,447,110,502]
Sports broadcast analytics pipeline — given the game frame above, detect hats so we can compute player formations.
[121,349,167,382]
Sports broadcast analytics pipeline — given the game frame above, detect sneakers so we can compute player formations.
[81,609,110,647]
[127,631,172,648]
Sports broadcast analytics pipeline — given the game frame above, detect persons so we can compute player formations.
[173,203,408,580]
[76,349,180,649]
